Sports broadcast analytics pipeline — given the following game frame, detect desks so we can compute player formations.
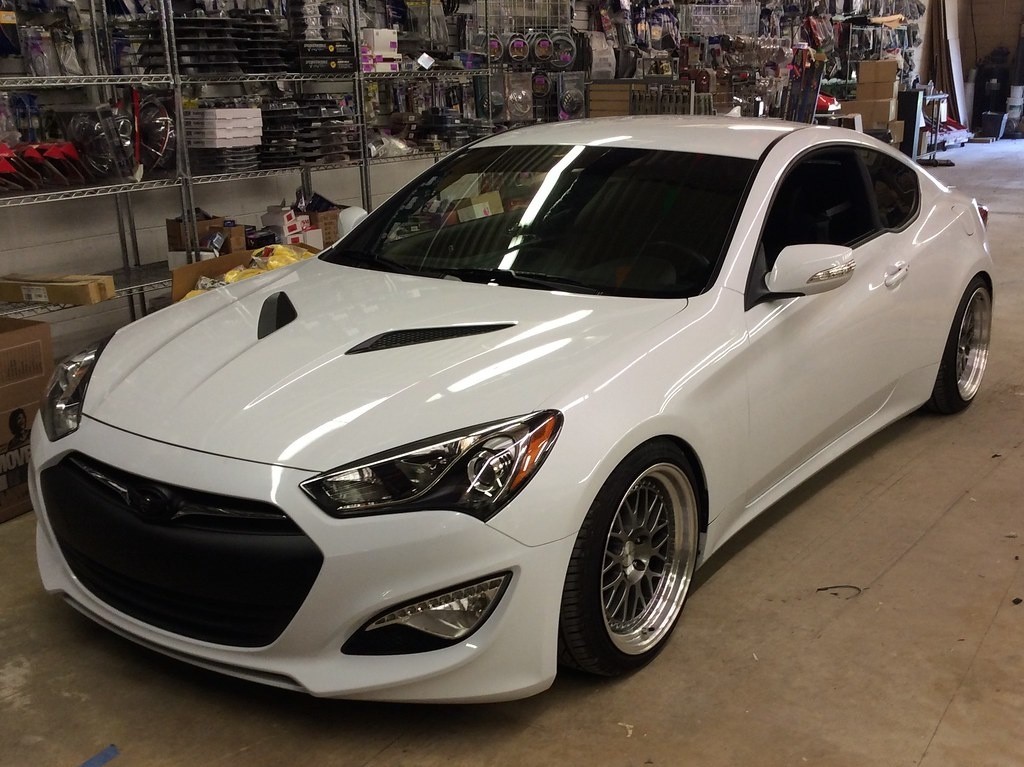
[916,91,954,167]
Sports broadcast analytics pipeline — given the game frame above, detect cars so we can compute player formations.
[25,114,995,709]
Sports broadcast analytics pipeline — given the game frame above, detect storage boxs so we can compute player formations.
[856,60,897,82]
[855,80,899,99]
[0,272,115,304]
[841,100,898,129]
[163,203,365,297]
[862,121,903,142]
[0,315,54,523]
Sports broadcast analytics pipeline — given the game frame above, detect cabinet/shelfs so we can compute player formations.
[0,0,192,365]
[291,0,491,215]
[100,0,367,315]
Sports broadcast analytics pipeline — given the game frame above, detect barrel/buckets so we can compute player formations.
[1011,86,1022,98]
[1007,98,1023,118]
